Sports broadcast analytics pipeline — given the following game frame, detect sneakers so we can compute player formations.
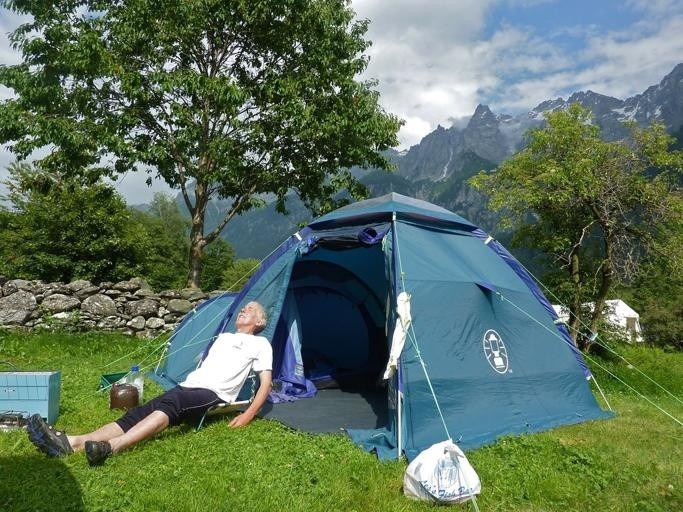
[85,439,112,467]
[27,413,74,458]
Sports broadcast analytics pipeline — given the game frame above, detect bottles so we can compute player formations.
[126,366,144,406]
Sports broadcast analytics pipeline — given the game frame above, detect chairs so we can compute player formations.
[196,368,260,431]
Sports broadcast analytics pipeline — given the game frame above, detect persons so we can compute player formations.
[26,300,274,466]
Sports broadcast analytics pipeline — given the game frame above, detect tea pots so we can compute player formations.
[110,381,139,412]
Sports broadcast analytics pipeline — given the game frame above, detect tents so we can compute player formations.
[150,191,616,461]
[551,304,570,322]
[580,299,645,343]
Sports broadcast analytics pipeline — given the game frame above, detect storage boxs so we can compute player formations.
[0,371,61,426]
